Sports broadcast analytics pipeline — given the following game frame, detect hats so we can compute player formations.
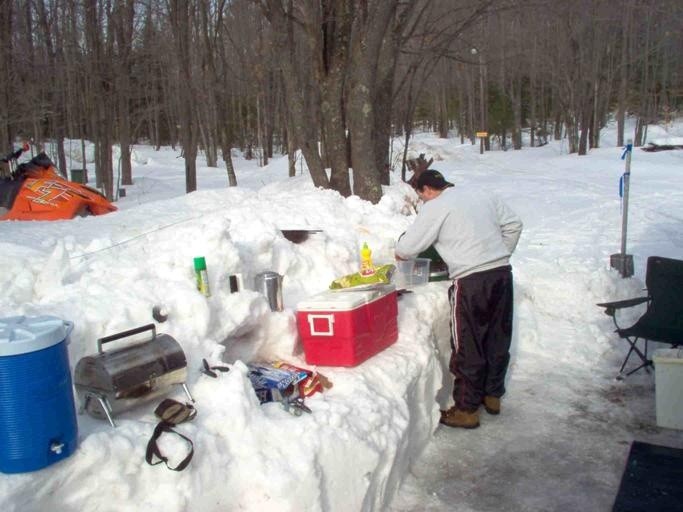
[417,170,454,189]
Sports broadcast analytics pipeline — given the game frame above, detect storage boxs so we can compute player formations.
[297,285,399,366]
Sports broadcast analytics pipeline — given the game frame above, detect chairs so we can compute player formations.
[596,256,683,378]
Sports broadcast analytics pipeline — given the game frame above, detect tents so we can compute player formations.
[0,152,117,222]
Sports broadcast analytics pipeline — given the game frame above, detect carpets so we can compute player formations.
[611,441,683,512]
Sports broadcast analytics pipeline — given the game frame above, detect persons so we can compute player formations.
[396,170,522,429]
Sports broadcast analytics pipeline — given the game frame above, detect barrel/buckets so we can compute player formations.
[0,314,78,474]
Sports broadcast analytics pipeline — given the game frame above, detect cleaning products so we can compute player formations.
[360,241,375,276]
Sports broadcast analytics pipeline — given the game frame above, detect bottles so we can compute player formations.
[254,270,284,313]
[358,242,376,278]
[193,256,211,297]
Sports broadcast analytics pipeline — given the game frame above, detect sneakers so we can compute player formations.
[483,394,500,414]
[439,406,481,429]
